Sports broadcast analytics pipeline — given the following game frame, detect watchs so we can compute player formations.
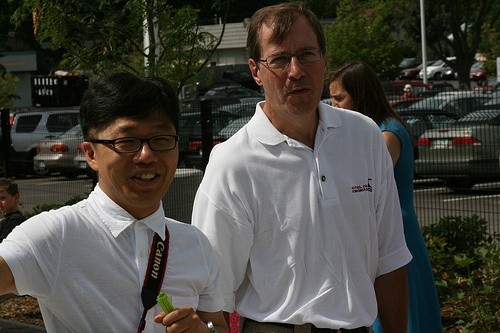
[206,320,217,333]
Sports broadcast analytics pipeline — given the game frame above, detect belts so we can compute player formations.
[245,316,370,333]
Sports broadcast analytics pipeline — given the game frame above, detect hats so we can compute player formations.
[474,53,483,61]
[404,84,412,92]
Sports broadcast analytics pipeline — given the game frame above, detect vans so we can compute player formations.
[9,109,84,176]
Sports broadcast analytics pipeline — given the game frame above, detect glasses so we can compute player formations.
[87,134,181,154]
[256,46,325,70]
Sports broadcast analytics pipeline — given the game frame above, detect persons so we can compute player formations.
[191,3,413,333]
[0,70,232,333]
[0,177,28,244]
[328,60,443,333]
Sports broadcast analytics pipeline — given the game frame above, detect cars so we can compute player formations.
[164,61,265,172]
[32,123,84,177]
[379,53,500,189]
[74,141,95,179]
